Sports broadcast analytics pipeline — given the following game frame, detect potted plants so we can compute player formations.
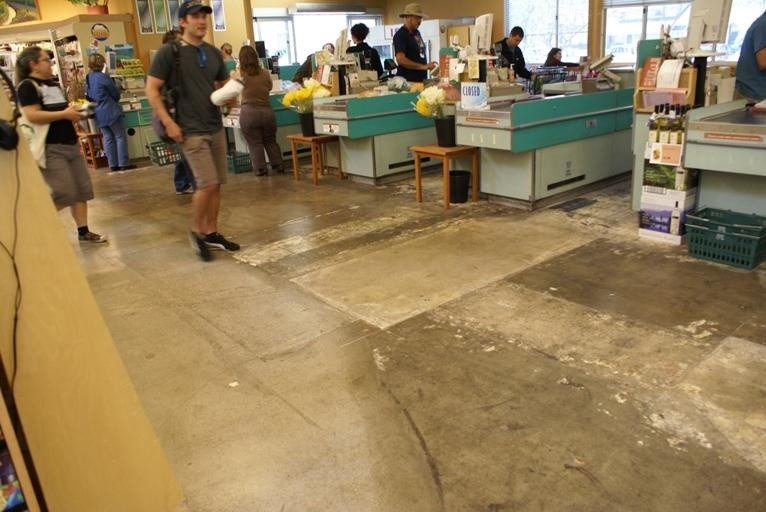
[69,0,109,14]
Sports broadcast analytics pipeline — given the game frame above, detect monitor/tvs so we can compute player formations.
[589,54,622,82]
[336,28,354,64]
[473,12,498,59]
[106,51,123,77]
[681,0,734,57]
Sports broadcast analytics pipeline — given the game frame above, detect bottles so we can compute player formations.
[646,103,701,144]
[675,156,686,190]
[669,201,680,236]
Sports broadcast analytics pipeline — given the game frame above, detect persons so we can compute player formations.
[144,0,241,261]
[291,43,335,88]
[162,29,194,195]
[733,11,766,102]
[86,53,137,171]
[15,46,107,245]
[219,43,236,72]
[490,26,535,81]
[542,48,579,67]
[393,3,438,83]
[238,45,285,177]
[345,23,384,79]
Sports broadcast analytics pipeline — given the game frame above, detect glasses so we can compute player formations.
[39,56,50,62]
[197,46,208,70]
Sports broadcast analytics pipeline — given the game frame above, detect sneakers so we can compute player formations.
[176,185,194,195]
[204,232,240,251]
[78,232,106,243]
[188,230,209,261]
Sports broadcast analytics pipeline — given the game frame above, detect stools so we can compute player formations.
[80,133,106,168]
[288,132,344,185]
[409,144,481,208]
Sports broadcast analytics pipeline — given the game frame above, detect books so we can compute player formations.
[656,59,686,89]
[640,57,662,89]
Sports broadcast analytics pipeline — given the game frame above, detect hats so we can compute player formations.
[179,1,212,16]
[399,3,429,19]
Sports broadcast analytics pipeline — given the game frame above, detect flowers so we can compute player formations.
[281,78,331,112]
[415,87,460,116]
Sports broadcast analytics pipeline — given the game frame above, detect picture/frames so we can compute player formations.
[167,0,182,31]
[151,0,170,34]
[136,0,154,34]
[210,1,226,31]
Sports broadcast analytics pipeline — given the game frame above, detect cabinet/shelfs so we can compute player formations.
[55,35,87,85]
[0,40,59,98]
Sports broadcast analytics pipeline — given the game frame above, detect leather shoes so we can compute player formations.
[111,167,119,171]
[120,164,136,170]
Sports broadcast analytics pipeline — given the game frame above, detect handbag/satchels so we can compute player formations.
[152,42,176,144]
[15,79,50,169]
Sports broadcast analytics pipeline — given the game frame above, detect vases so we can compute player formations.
[436,114,456,148]
[299,112,315,136]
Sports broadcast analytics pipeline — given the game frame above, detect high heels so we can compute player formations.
[256,167,269,176]
[278,165,285,174]
